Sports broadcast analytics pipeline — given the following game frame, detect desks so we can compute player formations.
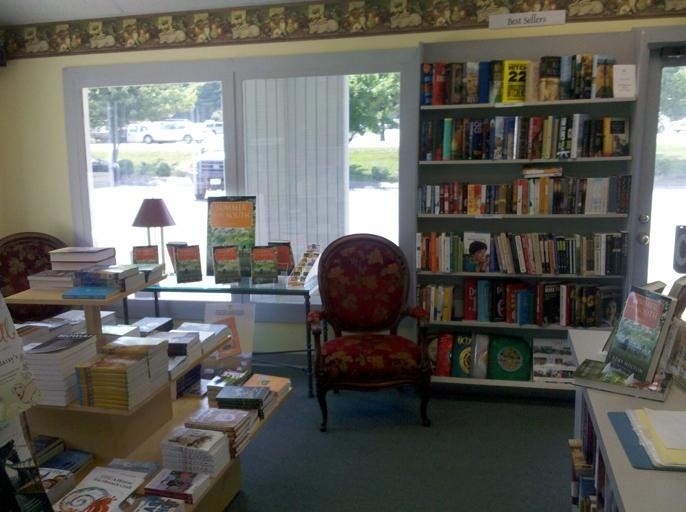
[124,269,330,398]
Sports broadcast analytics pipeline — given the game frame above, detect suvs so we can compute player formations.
[193,127,226,200]
[138,119,204,144]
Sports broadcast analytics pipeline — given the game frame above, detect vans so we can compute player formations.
[125,121,143,143]
[202,120,224,134]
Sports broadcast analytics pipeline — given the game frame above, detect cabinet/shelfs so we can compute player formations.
[0,271,293,512]
[416,31,643,392]
[567,329,686,512]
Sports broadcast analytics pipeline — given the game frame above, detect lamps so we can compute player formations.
[132,198,175,273]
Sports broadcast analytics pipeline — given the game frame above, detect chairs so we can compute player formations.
[310,233,431,433]
[0,232,78,323]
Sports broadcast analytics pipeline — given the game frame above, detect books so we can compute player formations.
[421,53,638,105]
[415,230,626,279]
[419,113,630,161]
[414,276,620,330]
[14,308,276,511]
[419,328,578,386]
[418,165,633,215]
[25,246,276,302]
[572,275,686,401]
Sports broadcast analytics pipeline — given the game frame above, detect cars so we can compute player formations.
[89,119,125,143]
